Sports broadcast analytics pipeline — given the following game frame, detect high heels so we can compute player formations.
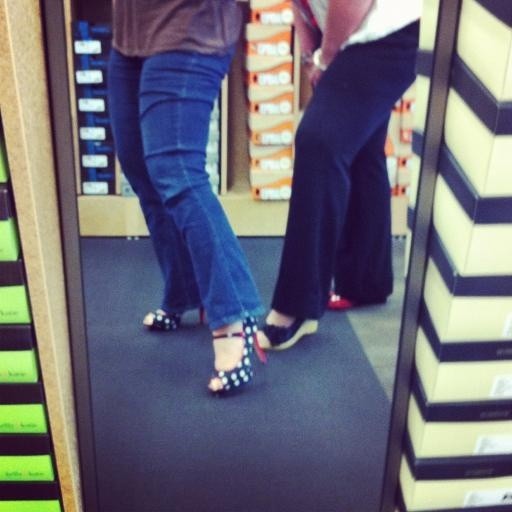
[258,314,320,351]
[208,313,267,394]
[141,304,206,331]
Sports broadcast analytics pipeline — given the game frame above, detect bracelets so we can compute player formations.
[311,47,328,73]
[299,49,316,66]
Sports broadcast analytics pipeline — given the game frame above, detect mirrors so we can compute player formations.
[40,0,462,512]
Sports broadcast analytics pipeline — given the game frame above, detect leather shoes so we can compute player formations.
[326,290,388,311]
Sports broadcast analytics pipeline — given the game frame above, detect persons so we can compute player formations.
[104,0,269,393]
[255,1,422,354]
